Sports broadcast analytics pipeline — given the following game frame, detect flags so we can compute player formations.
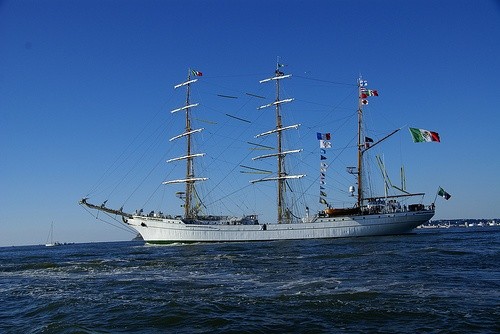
[318,140,332,149]
[408,127,441,143]
[437,186,452,201]
[361,99,369,105]
[360,80,379,99]
[316,132,332,140]
[320,162,329,172]
[318,190,328,205]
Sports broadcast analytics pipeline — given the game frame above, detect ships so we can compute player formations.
[78,56,452,243]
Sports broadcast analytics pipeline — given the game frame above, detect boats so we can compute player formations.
[45,219,60,248]
[417,219,500,229]
